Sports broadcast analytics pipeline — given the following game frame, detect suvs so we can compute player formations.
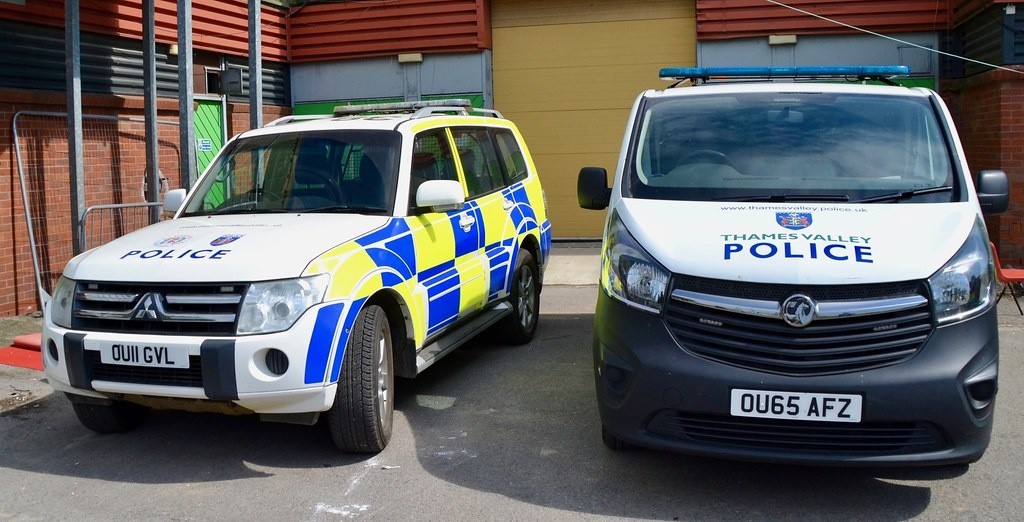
[41,98,552,455]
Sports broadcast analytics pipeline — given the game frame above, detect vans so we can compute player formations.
[578,62,1010,479]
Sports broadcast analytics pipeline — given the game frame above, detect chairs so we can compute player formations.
[442,148,481,197]
[414,153,439,180]
[349,153,391,210]
[291,155,339,211]
[989,241,1024,315]
